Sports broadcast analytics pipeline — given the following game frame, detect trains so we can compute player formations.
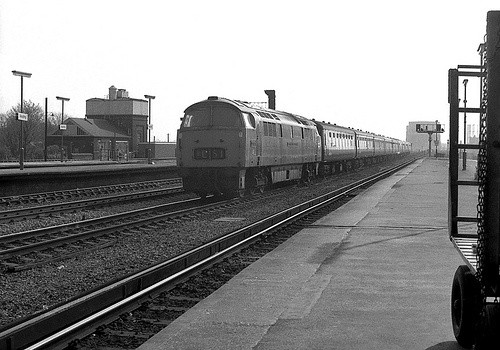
[175,90,413,205]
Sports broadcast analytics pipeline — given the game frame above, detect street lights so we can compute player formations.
[12,71,32,170]
[462,79,469,169]
[435,120,438,158]
[56,96,70,162]
[144,94,156,157]
